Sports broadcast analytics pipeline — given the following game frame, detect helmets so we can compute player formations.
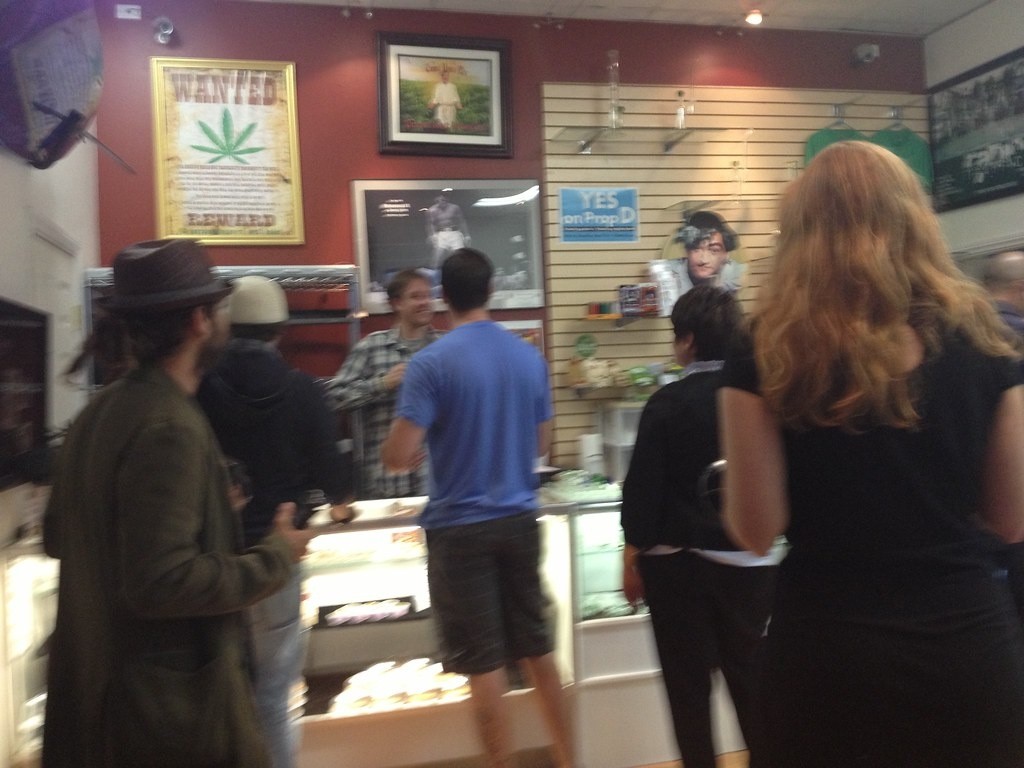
[227,277,288,326]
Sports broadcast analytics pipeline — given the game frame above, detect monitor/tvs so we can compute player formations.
[350,179,546,314]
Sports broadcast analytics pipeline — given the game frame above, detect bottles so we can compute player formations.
[674,89,686,129]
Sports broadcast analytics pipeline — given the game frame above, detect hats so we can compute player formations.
[95,239,234,313]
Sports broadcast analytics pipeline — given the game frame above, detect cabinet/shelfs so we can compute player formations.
[288,396,749,767]
[82,262,362,495]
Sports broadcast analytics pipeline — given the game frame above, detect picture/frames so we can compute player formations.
[149,53,306,244]
[349,178,546,316]
[375,29,513,160]
[926,46,1024,218]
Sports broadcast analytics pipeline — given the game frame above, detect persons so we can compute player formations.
[982,251,1024,339]
[620,285,786,768]
[429,70,463,127]
[714,141,1024,768]
[325,269,443,500]
[673,210,738,295]
[193,275,334,768]
[425,195,471,269]
[38,239,318,768]
[381,247,578,768]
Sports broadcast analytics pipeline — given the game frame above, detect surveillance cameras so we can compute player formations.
[857,45,881,62]
